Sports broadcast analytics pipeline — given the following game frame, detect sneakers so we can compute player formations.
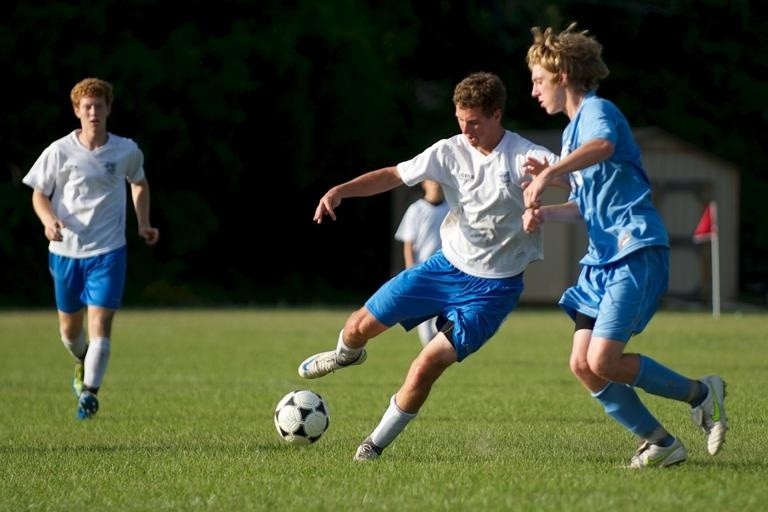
[353,437,383,460]
[616,436,686,467]
[298,348,367,379]
[72,359,98,419]
[692,375,726,455]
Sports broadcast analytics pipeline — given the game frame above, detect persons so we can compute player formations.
[22,77,159,420]
[394,180,450,347]
[296,73,569,462]
[522,21,729,468]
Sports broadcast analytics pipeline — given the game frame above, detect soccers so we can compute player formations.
[274,390,330,445]
